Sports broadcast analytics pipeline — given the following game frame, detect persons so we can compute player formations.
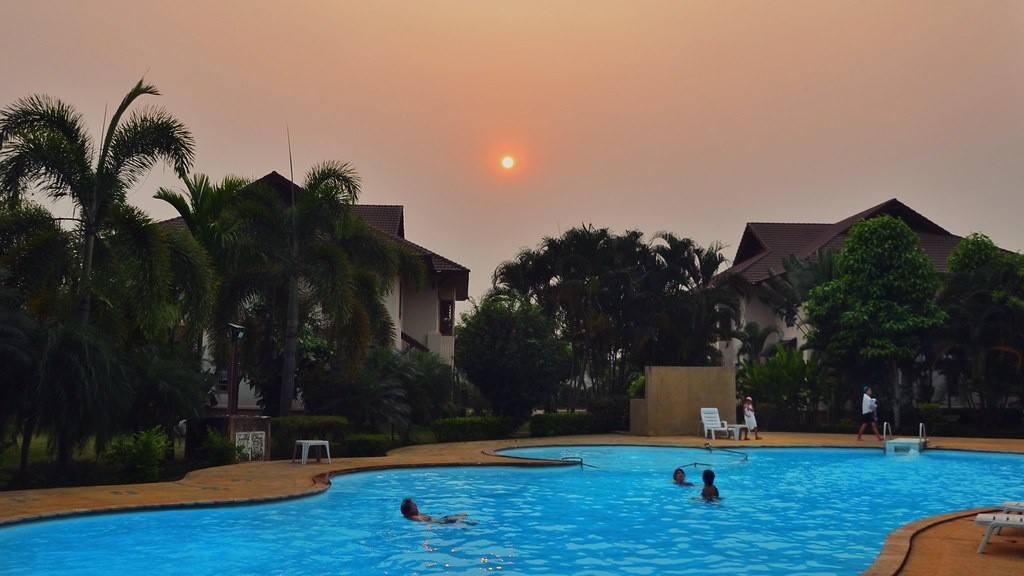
[743,396,762,440]
[857,386,884,441]
[702,470,719,503]
[673,468,693,489]
[401,498,479,527]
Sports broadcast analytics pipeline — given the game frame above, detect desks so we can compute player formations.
[727,424,752,441]
[292,440,333,465]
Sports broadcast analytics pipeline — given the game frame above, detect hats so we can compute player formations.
[863,386,869,393]
[746,397,752,401]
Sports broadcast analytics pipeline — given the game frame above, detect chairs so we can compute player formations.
[994,501,1024,536]
[700,407,736,440]
[974,513,1024,554]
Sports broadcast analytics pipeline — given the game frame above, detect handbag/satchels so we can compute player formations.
[858,412,872,424]
